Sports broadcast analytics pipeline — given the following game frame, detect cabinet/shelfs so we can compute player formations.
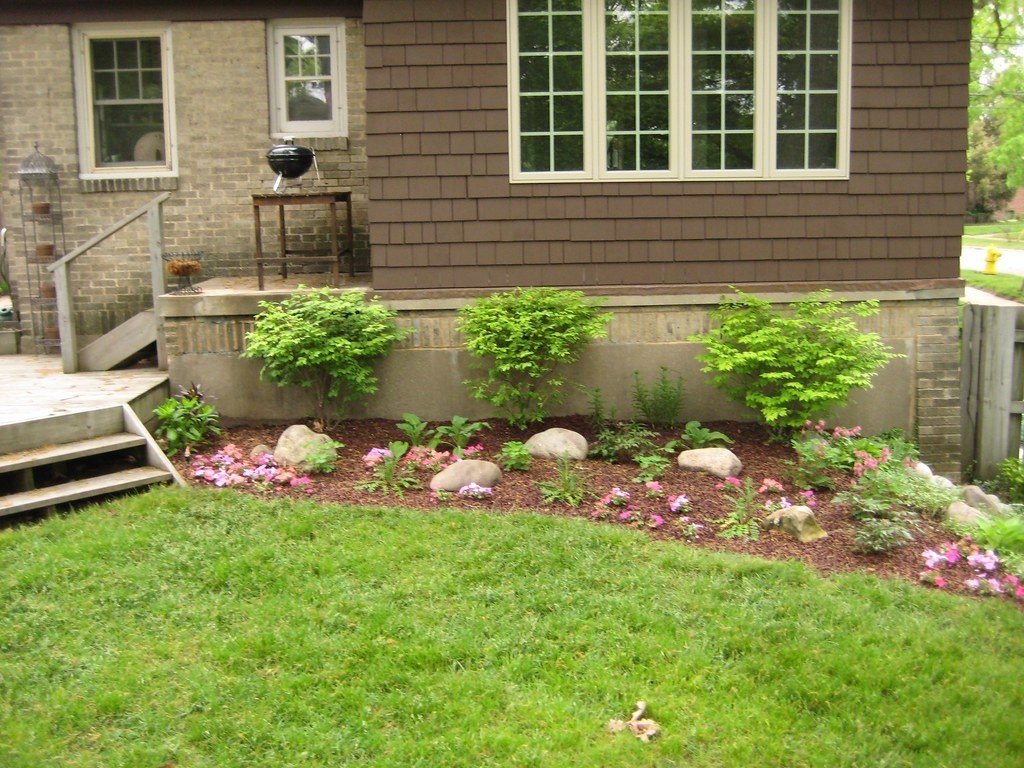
[16,142,68,359]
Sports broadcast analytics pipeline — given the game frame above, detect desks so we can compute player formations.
[252,191,353,290]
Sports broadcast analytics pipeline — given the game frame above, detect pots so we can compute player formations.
[267,139,314,179]
